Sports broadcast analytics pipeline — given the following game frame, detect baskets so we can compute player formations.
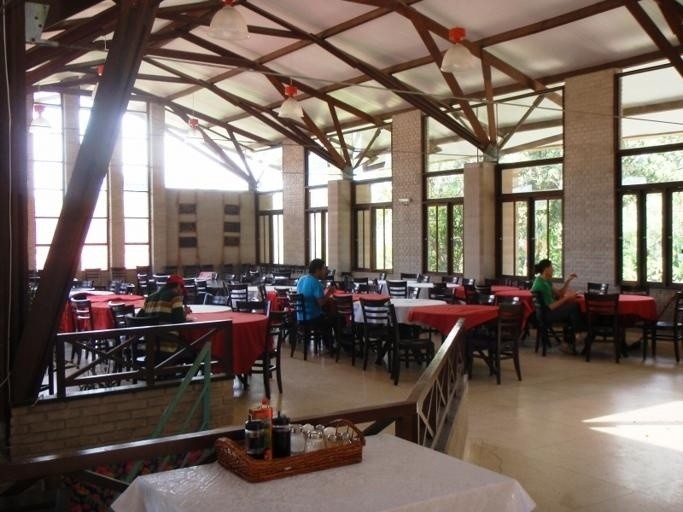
[215,419,366,484]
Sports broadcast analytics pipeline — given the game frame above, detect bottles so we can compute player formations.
[244,398,361,462]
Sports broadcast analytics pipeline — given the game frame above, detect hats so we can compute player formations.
[167,274,187,296]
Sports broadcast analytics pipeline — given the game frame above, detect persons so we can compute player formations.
[138,275,195,363]
[533,259,583,351]
[296,259,346,355]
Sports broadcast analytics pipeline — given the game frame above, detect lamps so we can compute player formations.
[34,105,49,127]
[91,64,105,96]
[278,79,304,118]
[184,95,204,144]
[441,28,480,73]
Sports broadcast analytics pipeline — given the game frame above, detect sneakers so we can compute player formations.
[559,342,574,354]
[322,344,336,353]
[575,331,588,343]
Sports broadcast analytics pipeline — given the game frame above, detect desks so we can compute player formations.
[110,431,538,511]
